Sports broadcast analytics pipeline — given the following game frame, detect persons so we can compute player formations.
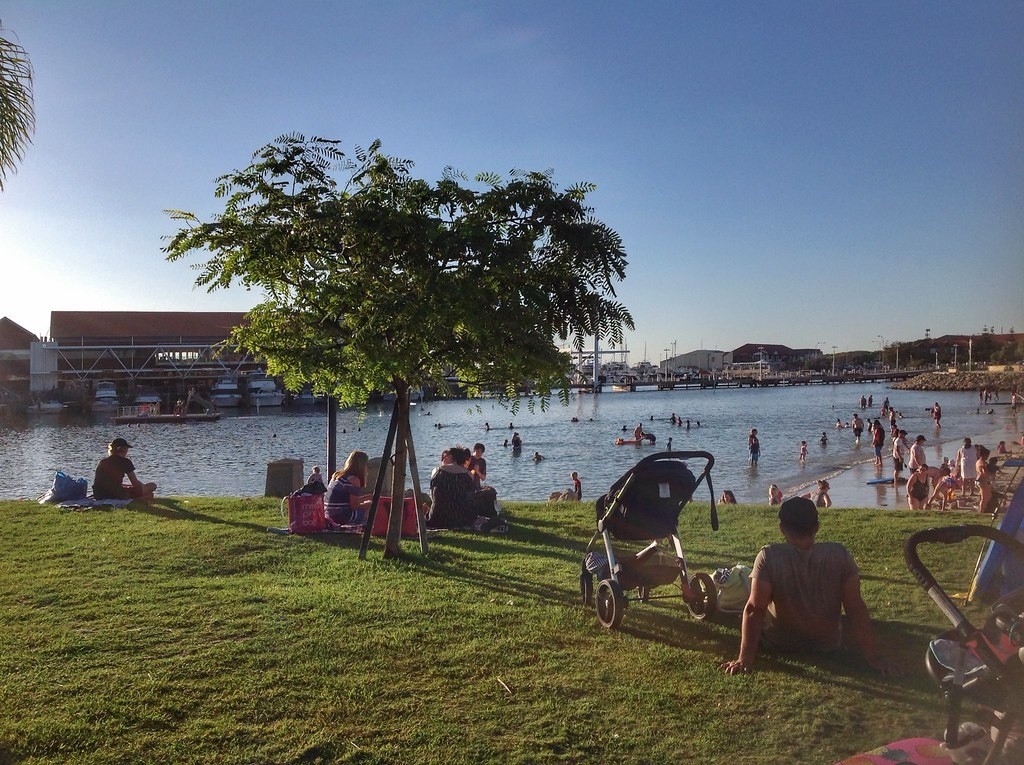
[308,467,323,484]
[533,452,543,460]
[91,438,157,502]
[323,451,372,524]
[801,481,831,507]
[820,432,827,445]
[571,472,581,500]
[718,496,903,679]
[670,413,690,429]
[634,422,656,444]
[429,444,507,533]
[667,438,672,452]
[748,428,760,466]
[799,441,807,461]
[512,431,521,452]
[836,419,841,429]
[850,386,1024,513]
[718,490,736,504]
[768,485,779,507]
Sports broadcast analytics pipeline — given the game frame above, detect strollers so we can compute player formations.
[580,451,717,629]
[903,525,1024,764]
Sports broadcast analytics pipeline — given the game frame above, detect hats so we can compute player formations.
[818,479,829,490]
[917,435,927,441]
[111,438,134,449]
[899,430,908,435]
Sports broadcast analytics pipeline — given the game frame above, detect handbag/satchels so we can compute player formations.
[38,473,87,506]
[710,567,754,614]
[371,496,417,538]
[280,495,325,534]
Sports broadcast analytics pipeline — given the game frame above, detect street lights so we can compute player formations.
[872,335,885,370]
[832,346,837,375]
[953,344,958,368]
[814,342,820,366]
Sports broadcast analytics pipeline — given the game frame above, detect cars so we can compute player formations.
[599,362,771,383]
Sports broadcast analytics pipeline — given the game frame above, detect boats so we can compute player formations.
[24,367,424,424]
